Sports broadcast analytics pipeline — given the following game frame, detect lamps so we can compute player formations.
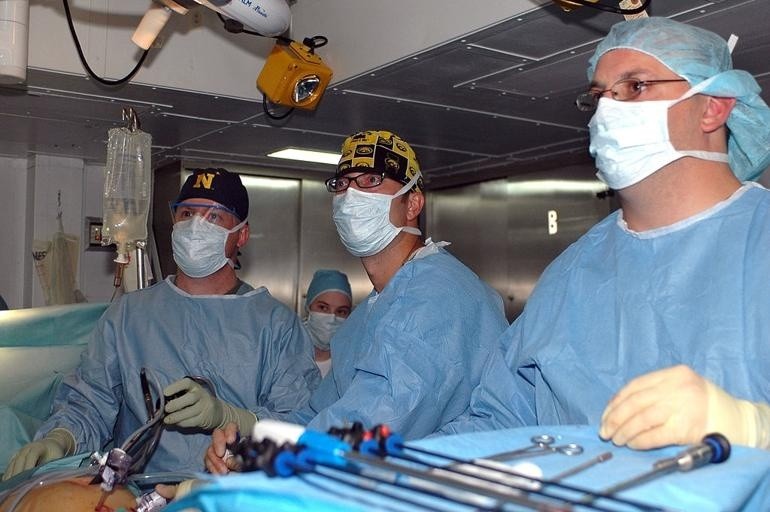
[255,41,334,115]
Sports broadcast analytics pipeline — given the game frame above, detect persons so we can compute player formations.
[0,168,325,481]
[300,270,352,378]
[419,17,770,451]
[205,131,511,478]
[0,453,142,512]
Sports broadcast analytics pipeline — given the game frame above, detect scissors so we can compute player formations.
[482,434,584,460]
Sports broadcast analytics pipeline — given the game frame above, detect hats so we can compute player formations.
[334,131,425,197]
[304,270,352,313]
[174,168,250,225]
[586,16,770,183]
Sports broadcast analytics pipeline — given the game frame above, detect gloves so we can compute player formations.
[599,364,770,452]
[1,428,78,480]
[163,378,258,438]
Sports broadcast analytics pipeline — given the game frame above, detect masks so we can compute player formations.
[587,76,682,190]
[304,305,346,352]
[331,171,422,257]
[171,213,249,278]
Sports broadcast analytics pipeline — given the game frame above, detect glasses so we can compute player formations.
[573,79,687,113]
[325,171,415,192]
